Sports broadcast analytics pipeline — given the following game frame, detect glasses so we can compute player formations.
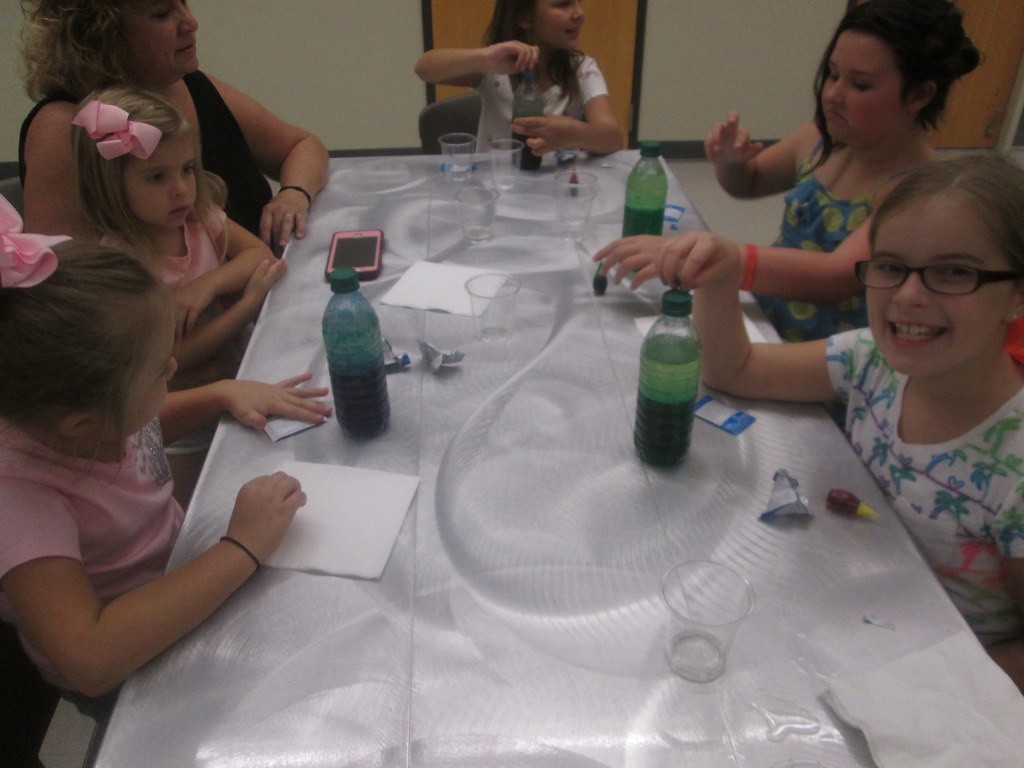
[855,260,1024,295]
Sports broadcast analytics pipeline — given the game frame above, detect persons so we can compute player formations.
[0,190,332,719]
[71,85,286,511]
[415,0,624,157]
[654,152,1024,694]
[591,0,981,427]
[18,0,329,246]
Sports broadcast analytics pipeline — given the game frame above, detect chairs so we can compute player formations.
[422,0,649,149]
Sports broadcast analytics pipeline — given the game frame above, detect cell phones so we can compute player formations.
[324,229,384,280]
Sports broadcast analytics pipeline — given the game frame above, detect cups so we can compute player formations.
[656,562,748,745]
[553,170,599,245]
[464,273,521,343]
[456,185,500,242]
[486,138,524,190]
[438,132,476,183]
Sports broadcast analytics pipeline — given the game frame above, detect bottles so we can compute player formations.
[633,288,701,467]
[592,262,608,294]
[322,266,392,439]
[826,488,879,519]
[511,67,544,171]
[621,140,668,239]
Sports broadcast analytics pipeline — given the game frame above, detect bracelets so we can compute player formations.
[220,536,261,568]
[280,186,312,202]
[742,243,757,290]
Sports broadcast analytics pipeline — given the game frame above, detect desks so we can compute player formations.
[95,147,1024,768]
